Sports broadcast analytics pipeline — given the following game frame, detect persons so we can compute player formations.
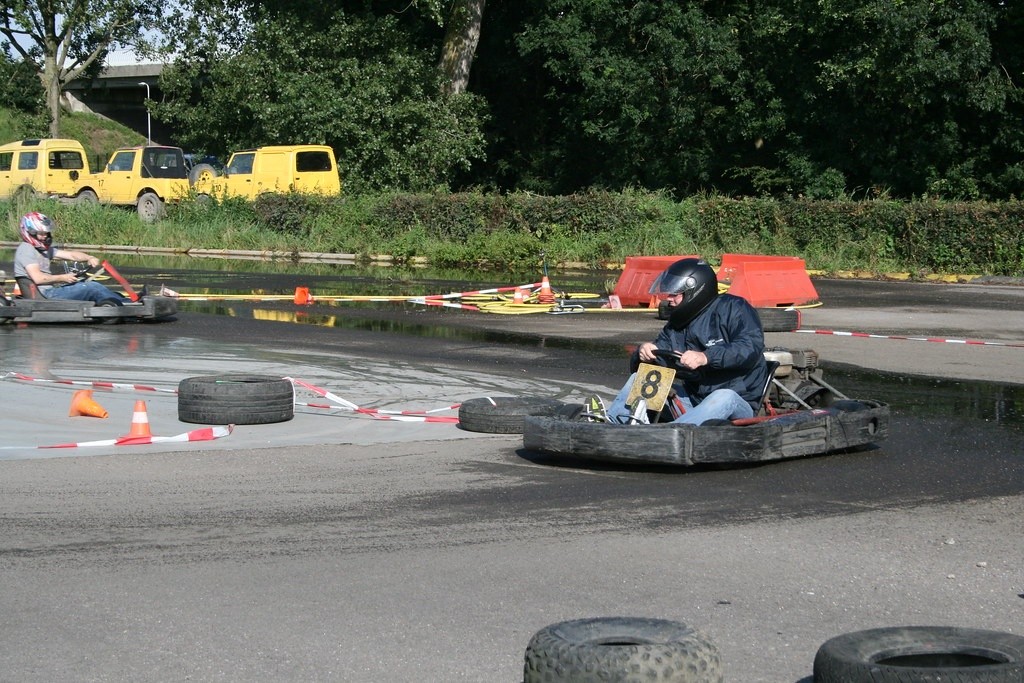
[583,258,769,428]
[14,211,149,306]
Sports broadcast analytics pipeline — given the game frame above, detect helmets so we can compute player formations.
[648,258,718,331]
[19,211,53,250]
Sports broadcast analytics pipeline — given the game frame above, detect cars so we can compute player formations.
[168,151,223,178]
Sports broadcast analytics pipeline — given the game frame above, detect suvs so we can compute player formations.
[223,146,343,207]
[0,135,96,211]
[88,143,228,223]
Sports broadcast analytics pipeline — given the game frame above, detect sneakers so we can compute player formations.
[584,394,609,422]
[137,283,148,304]
[628,395,650,424]
